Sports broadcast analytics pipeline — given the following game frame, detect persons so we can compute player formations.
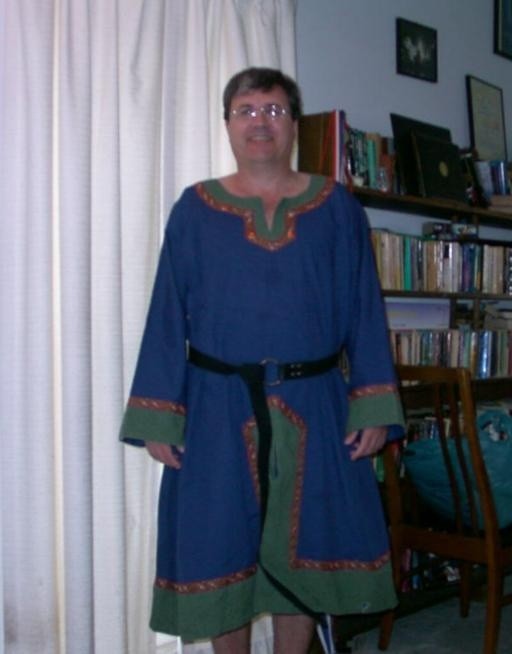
[115,66,409,653]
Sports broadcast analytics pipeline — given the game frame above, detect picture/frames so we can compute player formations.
[466,74,507,164]
[493,0,512,61]
[396,18,437,82]
[389,112,454,196]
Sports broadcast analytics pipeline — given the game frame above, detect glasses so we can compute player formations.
[224,103,294,121]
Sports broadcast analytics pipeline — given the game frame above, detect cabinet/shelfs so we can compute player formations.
[337,185,512,654]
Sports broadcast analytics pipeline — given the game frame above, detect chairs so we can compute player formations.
[377,365,510,653]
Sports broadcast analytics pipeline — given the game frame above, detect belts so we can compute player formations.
[180,347,348,383]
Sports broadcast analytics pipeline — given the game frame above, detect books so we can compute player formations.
[344,113,511,208]
[388,303,512,389]
[373,401,511,483]
[369,222,511,296]
